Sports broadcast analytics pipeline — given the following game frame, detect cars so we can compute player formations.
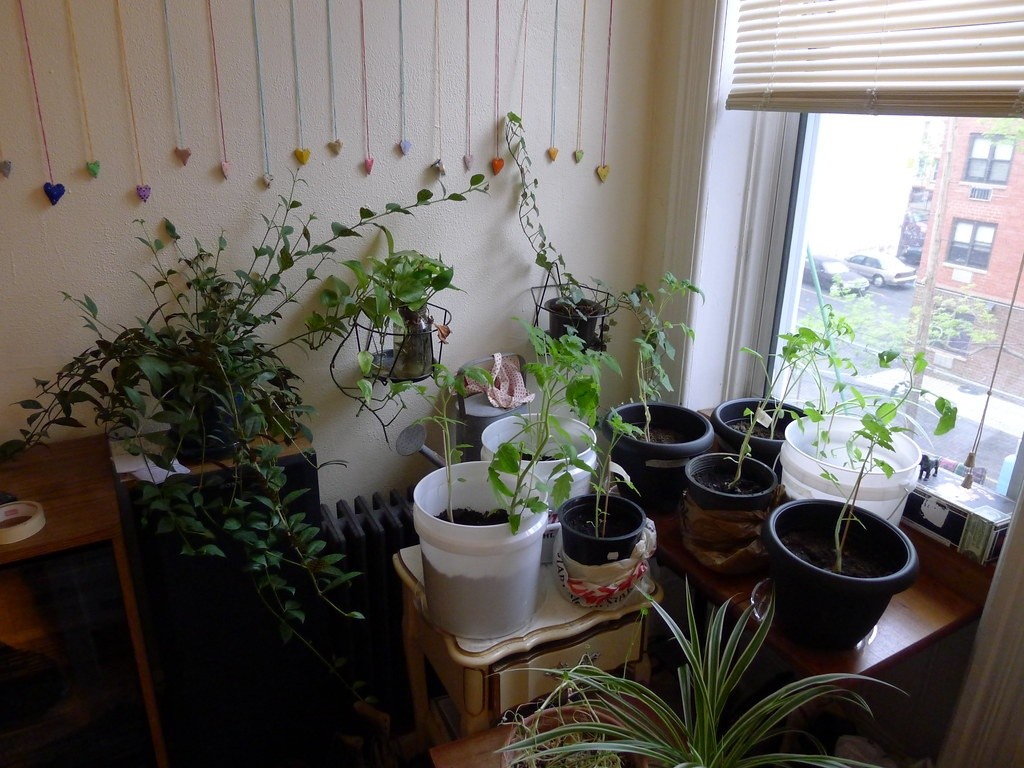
[803,255,870,296]
[902,212,927,235]
[840,252,916,288]
[901,247,922,265]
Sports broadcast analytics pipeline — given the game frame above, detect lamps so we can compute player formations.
[412,326,616,634]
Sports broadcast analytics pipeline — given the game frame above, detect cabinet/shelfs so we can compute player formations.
[121,428,320,768]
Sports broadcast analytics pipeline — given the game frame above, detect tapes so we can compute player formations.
[0,499,48,545]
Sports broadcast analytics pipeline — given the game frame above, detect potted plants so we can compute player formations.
[545,260,708,402]
[711,318,858,472]
[765,327,963,660]
[19,158,490,624]
[685,331,821,575]
[498,693,651,768]
[344,249,458,378]
[601,270,711,513]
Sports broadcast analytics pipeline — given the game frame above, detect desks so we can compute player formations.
[393,549,666,745]
[648,509,983,764]
[0,429,171,768]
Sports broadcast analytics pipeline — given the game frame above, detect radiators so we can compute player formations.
[316,485,429,661]
